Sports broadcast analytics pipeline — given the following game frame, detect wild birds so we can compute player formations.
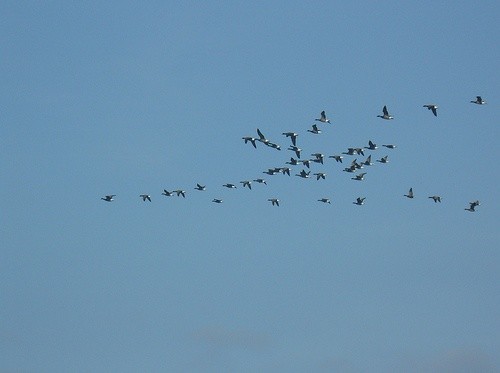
[382,144,398,150]
[423,105,439,117]
[352,197,366,206]
[313,172,326,181]
[194,184,207,191]
[376,105,395,120]
[351,173,367,181]
[222,184,238,189]
[253,179,267,185]
[242,128,281,151]
[376,156,389,163]
[342,140,380,156]
[306,124,322,134]
[139,194,152,202]
[470,96,487,105]
[464,201,480,212]
[100,195,116,202]
[212,198,224,203]
[262,167,292,176]
[329,154,344,163]
[285,152,325,168]
[161,189,186,198]
[287,145,302,159]
[268,199,280,207]
[315,111,332,124]
[295,170,311,178]
[239,180,252,190]
[428,196,441,203]
[403,188,414,199]
[342,154,374,173]
[317,199,331,205]
[282,132,299,146]
